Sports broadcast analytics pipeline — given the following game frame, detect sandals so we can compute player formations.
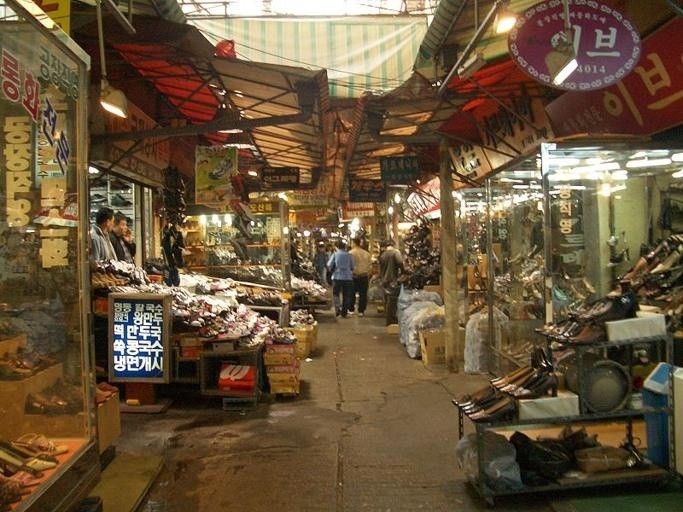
[9,429,68,453]
[1,469,38,487]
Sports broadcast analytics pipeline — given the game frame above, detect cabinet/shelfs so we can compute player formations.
[0,0,102,512]
[89,258,278,411]
[451,234,683,509]
[295,199,509,297]
[179,215,332,313]
[484,141,683,381]
[164,271,290,327]
[174,196,291,292]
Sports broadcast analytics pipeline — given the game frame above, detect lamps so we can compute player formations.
[213,93,244,133]
[223,131,254,150]
[94,0,128,121]
[544,1,579,86]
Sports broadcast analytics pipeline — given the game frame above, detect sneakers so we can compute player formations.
[93,256,314,346]
[111,193,132,206]
[357,311,364,317]
[215,247,327,298]
[348,310,354,314]
[90,192,105,203]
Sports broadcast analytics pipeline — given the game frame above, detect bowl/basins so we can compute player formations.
[578,359,634,418]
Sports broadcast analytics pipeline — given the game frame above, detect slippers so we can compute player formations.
[161,166,186,287]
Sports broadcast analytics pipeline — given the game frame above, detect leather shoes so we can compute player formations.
[584,301,605,322]
[462,393,496,410]
[96,388,112,398]
[1,442,57,464]
[26,389,62,414]
[0,492,19,504]
[44,386,76,414]
[452,385,491,405]
[464,397,504,414]
[570,322,607,343]
[96,393,106,403]
[458,387,496,406]
[540,320,570,337]
[96,382,117,392]
[0,344,58,380]
[579,299,603,319]
[533,324,552,331]
[2,480,29,495]
[547,319,571,339]
[593,298,636,319]
[468,397,514,420]
[490,376,503,383]
[548,319,592,344]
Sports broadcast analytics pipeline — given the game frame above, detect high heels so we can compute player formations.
[499,369,541,394]
[0,439,59,463]
[530,351,553,372]
[1,449,43,479]
[496,369,536,389]
[538,348,553,369]
[510,372,554,399]
[492,367,534,387]
[514,374,558,399]
[0,448,58,470]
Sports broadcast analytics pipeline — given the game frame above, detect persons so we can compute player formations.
[379,239,405,325]
[347,239,372,316]
[120,217,136,259]
[325,242,354,318]
[314,241,330,283]
[108,212,132,263]
[87,207,116,262]
